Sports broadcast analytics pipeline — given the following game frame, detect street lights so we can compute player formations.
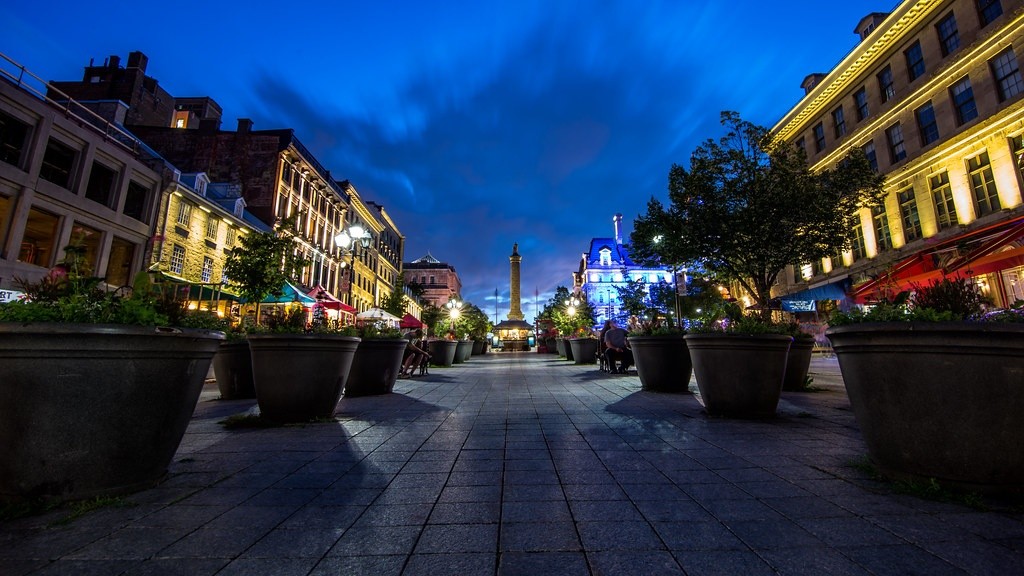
[332,215,372,305]
[652,232,682,327]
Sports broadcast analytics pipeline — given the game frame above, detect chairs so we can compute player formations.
[599,320,628,374]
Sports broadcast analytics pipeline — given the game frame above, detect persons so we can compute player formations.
[599,316,648,374]
[402,330,431,377]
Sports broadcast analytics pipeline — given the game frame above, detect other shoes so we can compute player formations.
[610,368,617,374]
[401,372,409,378]
[619,368,628,374]
[409,372,413,378]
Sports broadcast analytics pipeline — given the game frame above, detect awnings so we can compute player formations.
[747,280,848,311]
[161,273,246,305]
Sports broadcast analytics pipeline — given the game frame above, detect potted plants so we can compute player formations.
[213,326,256,399]
[683,310,793,419]
[825,269,1024,510]
[781,322,816,391]
[631,321,692,393]
[427,328,489,367]
[537,325,587,362]
[346,319,409,396]
[245,308,360,420]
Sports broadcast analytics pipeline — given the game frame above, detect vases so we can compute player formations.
[569,340,599,365]
[0,327,226,500]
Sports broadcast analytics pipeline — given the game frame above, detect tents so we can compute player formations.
[400,313,427,335]
[357,308,402,327]
[237,278,314,319]
[301,284,357,326]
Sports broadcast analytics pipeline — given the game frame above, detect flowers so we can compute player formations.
[0,228,175,326]
[568,332,599,340]
